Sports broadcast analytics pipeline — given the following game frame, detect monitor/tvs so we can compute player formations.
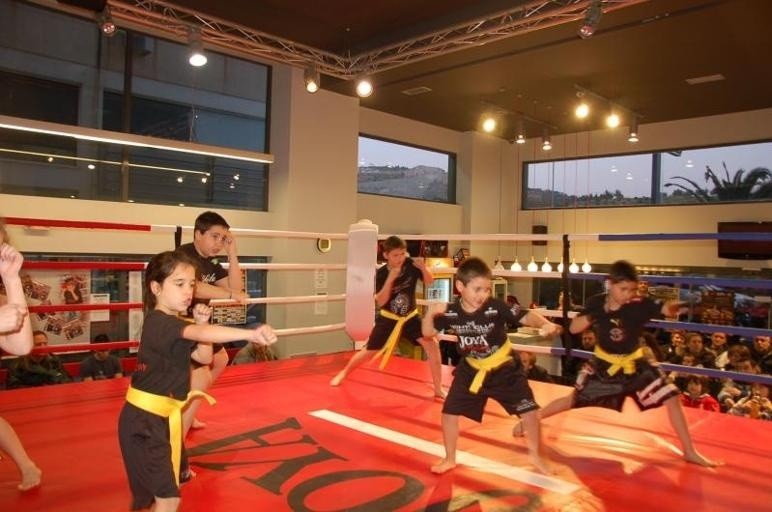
[717,221,772,260]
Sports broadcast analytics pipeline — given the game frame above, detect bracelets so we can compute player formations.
[229,290,232,300]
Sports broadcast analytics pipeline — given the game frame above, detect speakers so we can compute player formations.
[530,225,548,246]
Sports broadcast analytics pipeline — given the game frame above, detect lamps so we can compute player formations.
[576,1,614,39]
[479,100,560,156]
[353,71,373,99]
[567,83,647,146]
[92,1,120,40]
[303,57,322,96]
[186,22,208,67]
[494,114,593,277]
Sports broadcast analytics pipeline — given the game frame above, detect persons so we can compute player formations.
[175,211,250,445]
[512,260,717,468]
[118,250,279,512]
[7,331,72,389]
[79,334,124,382]
[329,236,449,401]
[0,221,42,491]
[421,257,564,477]
